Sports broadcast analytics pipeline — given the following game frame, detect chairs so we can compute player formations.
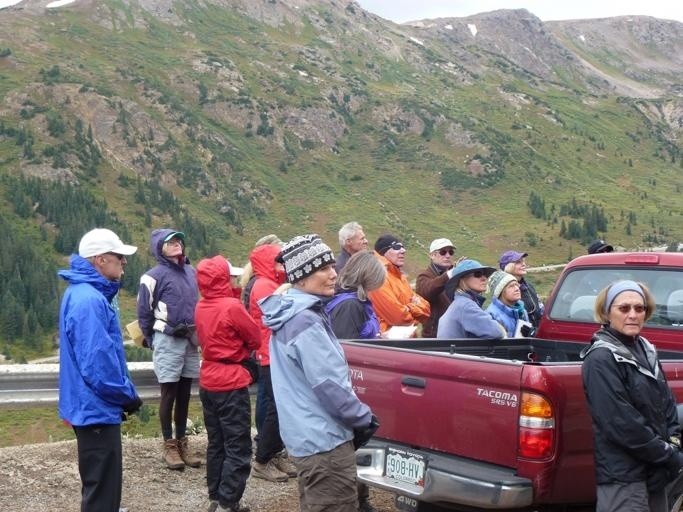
[667,289,683,326]
[569,295,602,324]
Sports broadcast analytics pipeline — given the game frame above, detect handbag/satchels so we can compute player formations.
[242,358,260,385]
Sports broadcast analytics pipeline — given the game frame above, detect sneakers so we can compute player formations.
[252,459,289,483]
[274,454,297,478]
[208,502,250,512]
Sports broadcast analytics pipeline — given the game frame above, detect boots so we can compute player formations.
[160,438,186,470]
[178,435,202,468]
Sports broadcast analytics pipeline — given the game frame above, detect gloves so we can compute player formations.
[354,415,380,452]
[520,325,536,337]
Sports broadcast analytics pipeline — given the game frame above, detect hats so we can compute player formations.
[429,237,456,253]
[280,232,337,285]
[498,249,529,271]
[444,258,497,300]
[164,231,186,242]
[587,239,614,254]
[225,259,244,277]
[77,228,138,259]
[488,269,518,300]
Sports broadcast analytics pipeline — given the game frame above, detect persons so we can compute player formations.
[581,280,682,511]
[136,229,203,469]
[57,228,143,512]
[241,234,298,482]
[321,250,387,512]
[334,221,614,341]
[257,234,380,512]
[195,254,261,512]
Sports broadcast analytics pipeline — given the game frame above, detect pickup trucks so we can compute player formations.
[336,243,682,511]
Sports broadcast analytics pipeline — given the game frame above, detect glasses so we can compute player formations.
[437,249,454,257]
[469,271,483,278]
[612,302,647,315]
[388,244,404,251]
